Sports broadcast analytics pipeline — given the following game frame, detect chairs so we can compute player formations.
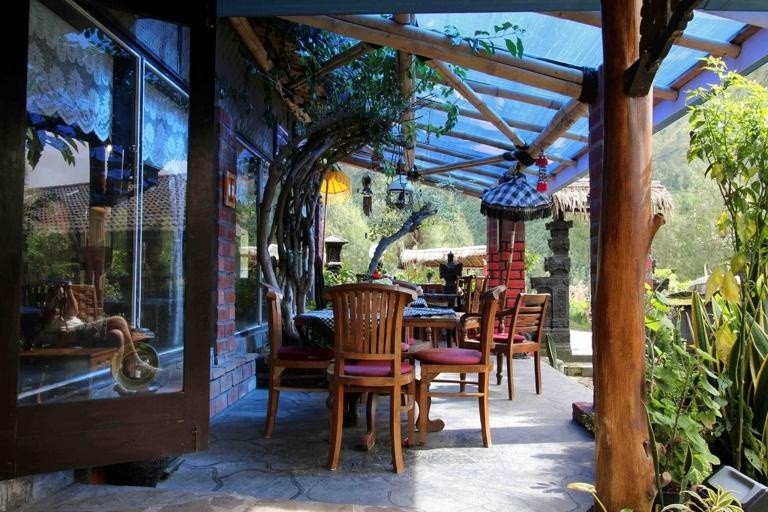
[415,298,496,450]
[459,293,551,402]
[321,283,418,474]
[356,275,490,356]
[264,290,333,441]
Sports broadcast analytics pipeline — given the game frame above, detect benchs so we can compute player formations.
[20,284,154,402]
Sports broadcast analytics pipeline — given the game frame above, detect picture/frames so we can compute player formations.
[224,170,236,209]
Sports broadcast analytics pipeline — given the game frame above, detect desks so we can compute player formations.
[295,309,458,452]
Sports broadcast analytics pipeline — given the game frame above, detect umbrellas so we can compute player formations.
[317,170,353,264]
[479,174,552,334]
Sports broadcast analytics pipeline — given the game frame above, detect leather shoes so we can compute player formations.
[111,384,136,396]
[128,362,161,374]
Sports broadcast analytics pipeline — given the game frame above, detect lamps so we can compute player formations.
[320,163,351,275]
[389,173,414,211]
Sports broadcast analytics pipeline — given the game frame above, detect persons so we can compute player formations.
[42,282,170,399]
